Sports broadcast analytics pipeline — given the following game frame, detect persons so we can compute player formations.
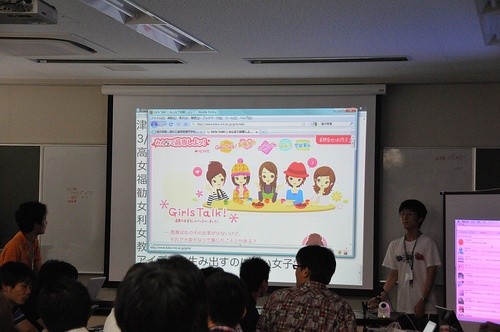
[0,255,270,332]
[0,201,48,274]
[368,199,441,332]
[256,244,357,332]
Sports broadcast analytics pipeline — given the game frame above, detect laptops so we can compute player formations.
[88,277,106,298]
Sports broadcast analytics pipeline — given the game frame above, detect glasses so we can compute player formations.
[292,264,307,269]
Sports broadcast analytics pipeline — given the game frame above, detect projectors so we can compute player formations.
[0,0,58,24]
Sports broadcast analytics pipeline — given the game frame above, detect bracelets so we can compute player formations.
[420,296,427,302]
